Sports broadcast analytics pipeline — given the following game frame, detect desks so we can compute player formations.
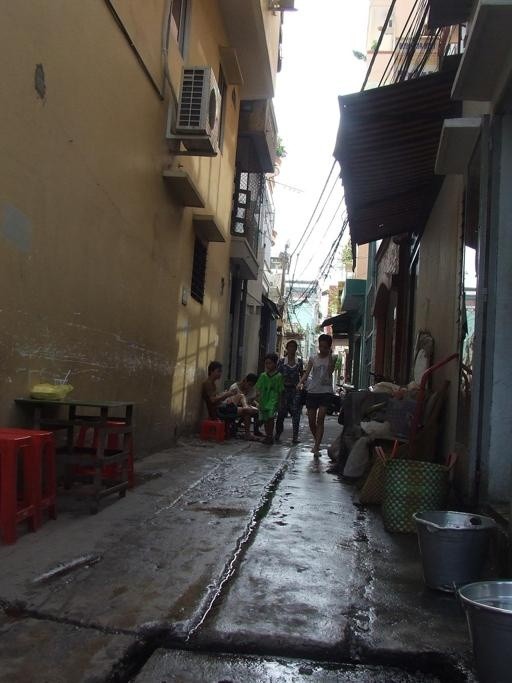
[14,389,142,516]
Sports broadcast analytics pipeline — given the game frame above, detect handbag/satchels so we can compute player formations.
[374,445,461,537]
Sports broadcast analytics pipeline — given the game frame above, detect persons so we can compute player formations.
[274,339,305,443]
[224,369,257,412]
[203,360,268,442]
[296,334,336,456]
[251,351,284,446]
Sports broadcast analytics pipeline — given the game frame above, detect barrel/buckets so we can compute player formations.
[450,580,512,683]
[413,510,496,594]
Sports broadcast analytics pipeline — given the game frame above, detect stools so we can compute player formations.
[0,423,134,544]
[200,419,226,443]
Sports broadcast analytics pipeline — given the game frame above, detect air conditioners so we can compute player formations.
[175,66,223,156]
